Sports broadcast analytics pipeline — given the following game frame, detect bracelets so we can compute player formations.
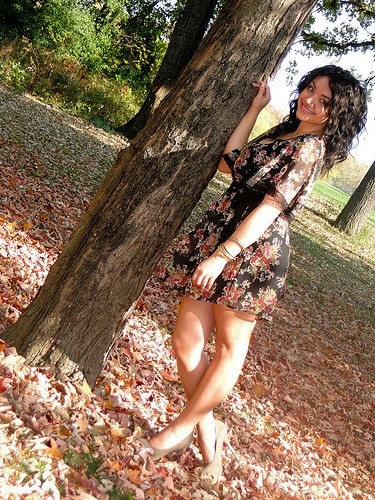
[229,236,244,253]
[218,243,239,263]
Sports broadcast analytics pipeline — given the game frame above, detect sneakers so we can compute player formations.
[138,431,193,461]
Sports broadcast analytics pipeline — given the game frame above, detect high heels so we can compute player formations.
[202,419,236,484]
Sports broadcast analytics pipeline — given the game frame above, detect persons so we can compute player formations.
[142,64,368,483]
